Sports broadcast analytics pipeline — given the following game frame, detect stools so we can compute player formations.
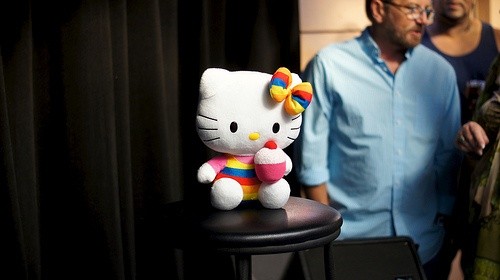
[168,194,342,279]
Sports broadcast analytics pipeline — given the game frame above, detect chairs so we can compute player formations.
[296,235,426,280]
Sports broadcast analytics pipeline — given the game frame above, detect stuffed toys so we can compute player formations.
[196,66,313,210]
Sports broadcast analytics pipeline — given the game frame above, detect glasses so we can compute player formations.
[386,0,434,20]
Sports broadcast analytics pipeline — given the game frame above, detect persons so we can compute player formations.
[297,0,462,280]
[420,0,500,126]
[457,54,500,280]
